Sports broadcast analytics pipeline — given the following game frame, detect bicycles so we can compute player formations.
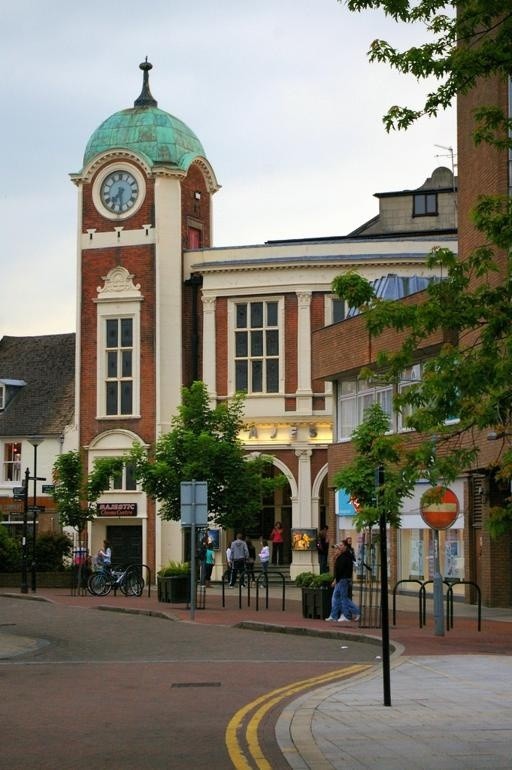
[88,563,146,598]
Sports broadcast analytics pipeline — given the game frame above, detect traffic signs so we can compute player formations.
[21,479,26,486]
[13,495,24,500]
[28,477,46,481]
[27,508,45,513]
[12,487,25,494]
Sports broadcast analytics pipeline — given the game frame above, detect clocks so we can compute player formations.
[99,168,142,215]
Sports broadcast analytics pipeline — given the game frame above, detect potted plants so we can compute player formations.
[294,570,338,620]
[155,560,192,604]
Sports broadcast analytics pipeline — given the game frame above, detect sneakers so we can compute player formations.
[325,614,360,621]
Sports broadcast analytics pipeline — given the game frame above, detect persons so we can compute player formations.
[324,536,362,624]
[97,539,113,578]
[270,520,288,565]
[202,531,271,589]
[61,539,93,598]
[315,524,330,575]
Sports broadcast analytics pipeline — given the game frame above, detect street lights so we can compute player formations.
[487,429,512,440]
[28,438,43,594]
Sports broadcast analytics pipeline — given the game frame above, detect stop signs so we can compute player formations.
[420,488,460,531]
